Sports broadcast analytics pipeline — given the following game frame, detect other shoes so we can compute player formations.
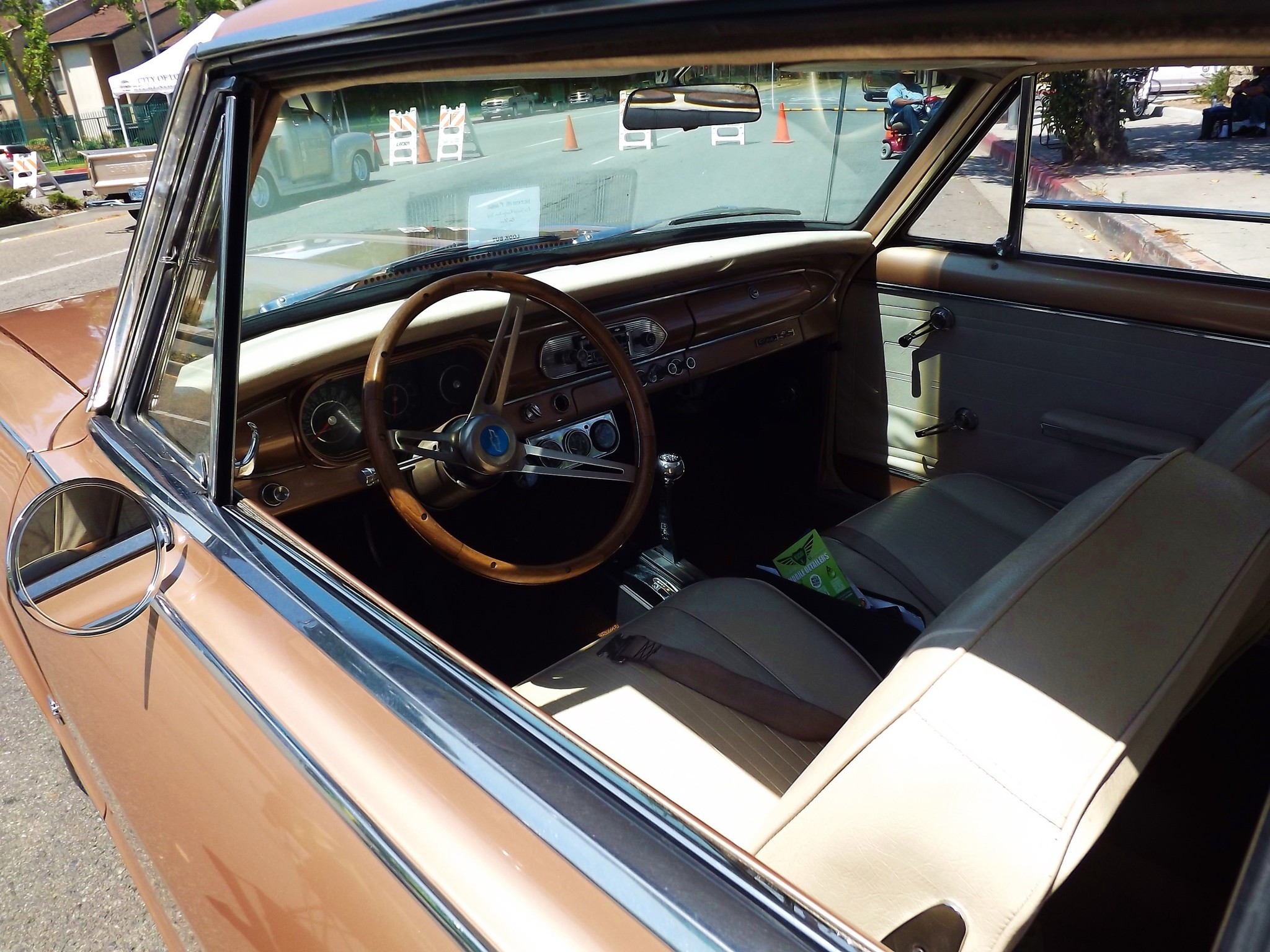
[1246,126,1267,137]
[914,132,921,138]
[1232,125,1256,136]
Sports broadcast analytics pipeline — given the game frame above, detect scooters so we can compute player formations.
[880,95,945,161]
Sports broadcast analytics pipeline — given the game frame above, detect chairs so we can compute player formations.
[512,382,1270,952]
[885,108,928,130]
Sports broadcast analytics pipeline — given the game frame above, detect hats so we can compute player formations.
[900,70,916,77]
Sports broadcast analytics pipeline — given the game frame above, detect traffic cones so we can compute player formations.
[369,131,389,166]
[771,101,795,144]
[561,114,582,152]
[416,127,434,164]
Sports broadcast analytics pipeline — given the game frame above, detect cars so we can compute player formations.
[0,0,1267,952]
[1117,66,1230,120]
[568,86,614,108]
[0,144,42,180]
[480,85,543,123]
[860,68,905,102]
[75,92,382,228]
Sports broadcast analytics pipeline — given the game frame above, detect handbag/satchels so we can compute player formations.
[1198,104,1233,140]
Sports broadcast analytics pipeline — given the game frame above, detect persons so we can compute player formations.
[1231,66,1270,137]
[887,69,930,138]
